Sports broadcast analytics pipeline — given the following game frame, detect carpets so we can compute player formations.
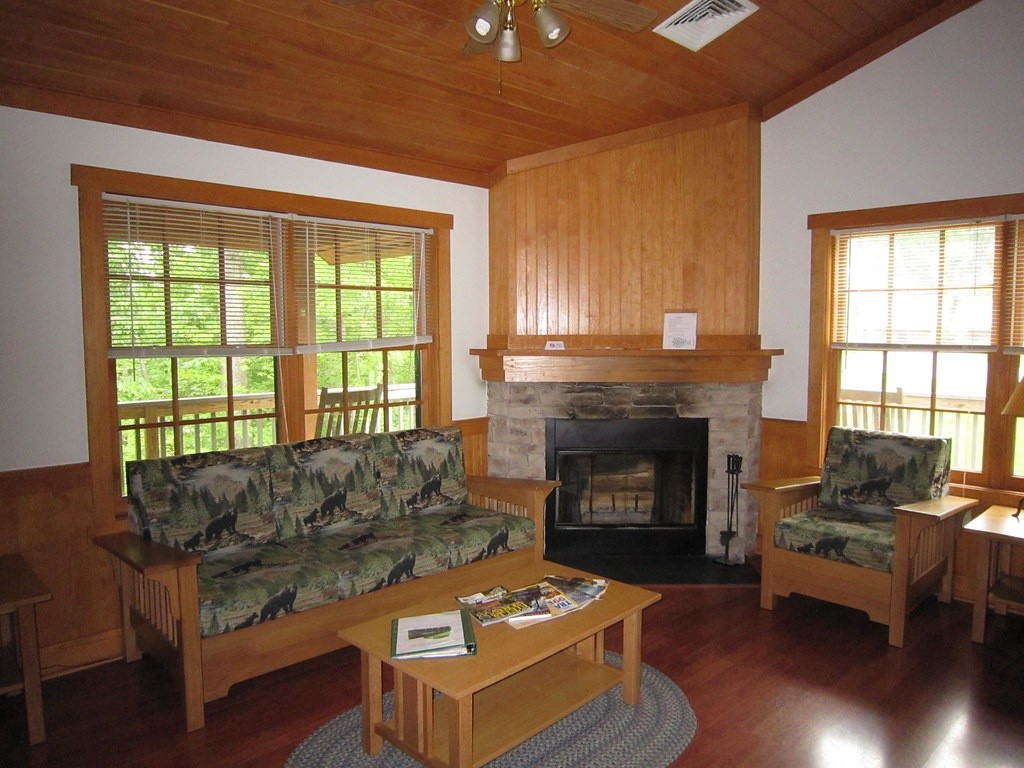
[284,648,699,768]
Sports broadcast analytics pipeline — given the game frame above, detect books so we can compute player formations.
[390,608,477,660]
[455,574,610,629]
[661,312,697,351]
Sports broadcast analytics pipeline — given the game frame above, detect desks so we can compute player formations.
[961,503,1024,643]
[336,560,662,768]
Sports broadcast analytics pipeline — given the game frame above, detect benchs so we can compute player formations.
[89,425,562,734]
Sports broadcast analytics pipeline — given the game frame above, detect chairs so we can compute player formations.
[740,426,979,648]
[314,383,383,438]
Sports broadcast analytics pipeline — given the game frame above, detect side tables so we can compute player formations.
[1,551,53,745]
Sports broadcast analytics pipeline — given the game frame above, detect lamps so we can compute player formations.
[464,0,571,63]
[1000,376,1024,517]
